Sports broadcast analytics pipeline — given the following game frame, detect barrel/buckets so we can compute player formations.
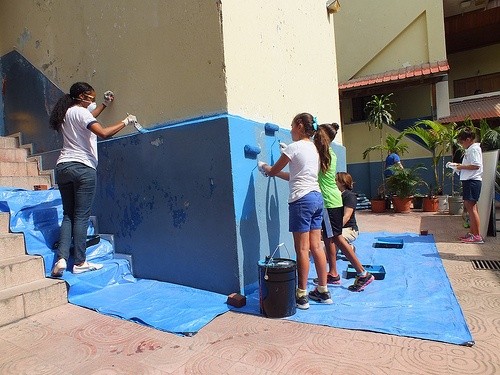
[257,243,297,318]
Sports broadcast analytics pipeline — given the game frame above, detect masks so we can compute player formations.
[75,93,97,113]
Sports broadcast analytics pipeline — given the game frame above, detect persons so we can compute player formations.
[456,128,485,243]
[52,81,137,278]
[278,121,375,292]
[385,152,404,178]
[334,172,359,260]
[258,113,333,309]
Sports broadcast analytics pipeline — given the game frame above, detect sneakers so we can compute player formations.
[313,272,341,284]
[72,262,103,274]
[459,232,484,244]
[51,258,67,277]
[295,294,310,309]
[308,287,331,303]
[347,270,375,292]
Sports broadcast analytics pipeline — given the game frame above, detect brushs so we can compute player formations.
[244,145,262,162]
[126,112,145,134]
[265,122,281,143]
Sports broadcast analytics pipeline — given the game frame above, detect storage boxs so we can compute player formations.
[377,239,404,250]
[345,264,386,281]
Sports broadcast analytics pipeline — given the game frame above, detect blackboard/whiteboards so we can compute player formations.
[477,150,499,242]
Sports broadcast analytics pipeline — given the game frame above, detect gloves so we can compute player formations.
[122,114,137,126]
[257,160,268,172]
[279,142,287,152]
[103,91,114,108]
[261,171,269,177]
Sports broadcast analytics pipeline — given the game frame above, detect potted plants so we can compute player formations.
[413,190,425,209]
[371,184,386,214]
[385,165,428,212]
[405,119,463,210]
[449,186,465,214]
[423,183,438,212]
